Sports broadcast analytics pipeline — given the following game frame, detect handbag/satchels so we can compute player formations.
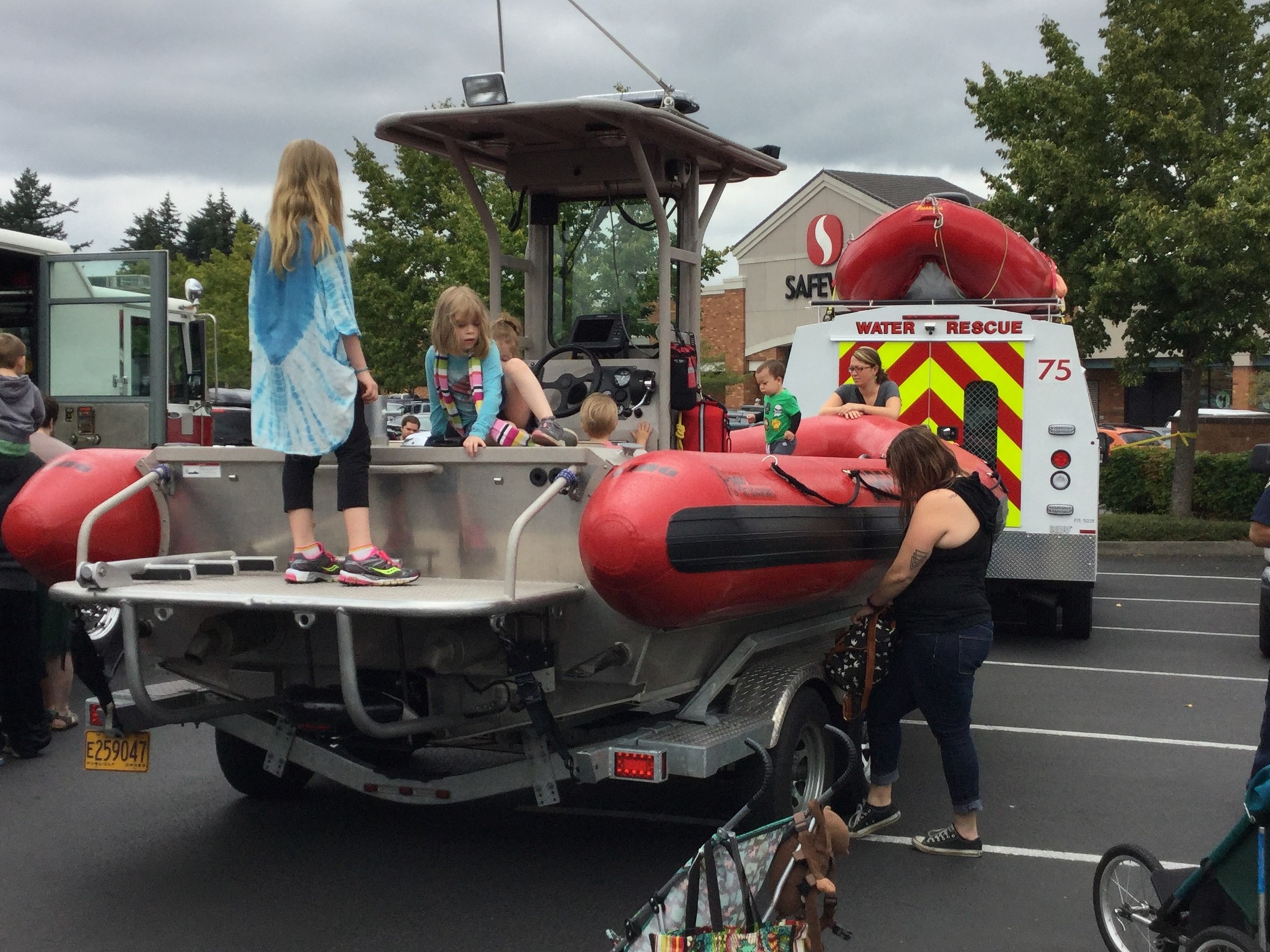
[825,606,900,722]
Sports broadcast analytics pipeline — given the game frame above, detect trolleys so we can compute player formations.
[610,723,857,952]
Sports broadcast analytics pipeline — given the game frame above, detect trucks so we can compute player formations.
[778,312,1104,642]
[0,227,220,663]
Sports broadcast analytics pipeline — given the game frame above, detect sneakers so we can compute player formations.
[910,821,982,857]
[284,542,346,581]
[338,546,421,586]
[561,427,578,445]
[532,417,564,445]
[846,800,900,837]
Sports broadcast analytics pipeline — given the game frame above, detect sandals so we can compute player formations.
[52,713,79,730]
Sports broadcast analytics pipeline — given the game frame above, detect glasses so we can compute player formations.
[848,365,874,373]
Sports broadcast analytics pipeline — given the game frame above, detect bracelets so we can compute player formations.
[355,367,369,374]
[867,594,883,610]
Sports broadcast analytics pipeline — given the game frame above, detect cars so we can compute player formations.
[385,394,432,436]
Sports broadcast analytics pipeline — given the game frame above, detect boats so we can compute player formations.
[833,197,1065,312]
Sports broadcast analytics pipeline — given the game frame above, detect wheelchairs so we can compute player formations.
[1093,762,1270,952]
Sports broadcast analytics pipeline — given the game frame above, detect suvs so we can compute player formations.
[1098,420,1171,459]
[207,388,251,447]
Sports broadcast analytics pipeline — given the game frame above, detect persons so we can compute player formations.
[248,138,422,586]
[397,415,420,440]
[1244,487,1270,787]
[754,398,760,405]
[0,333,76,768]
[425,286,579,456]
[819,348,902,420]
[579,392,653,457]
[746,359,801,455]
[848,426,1001,857]
